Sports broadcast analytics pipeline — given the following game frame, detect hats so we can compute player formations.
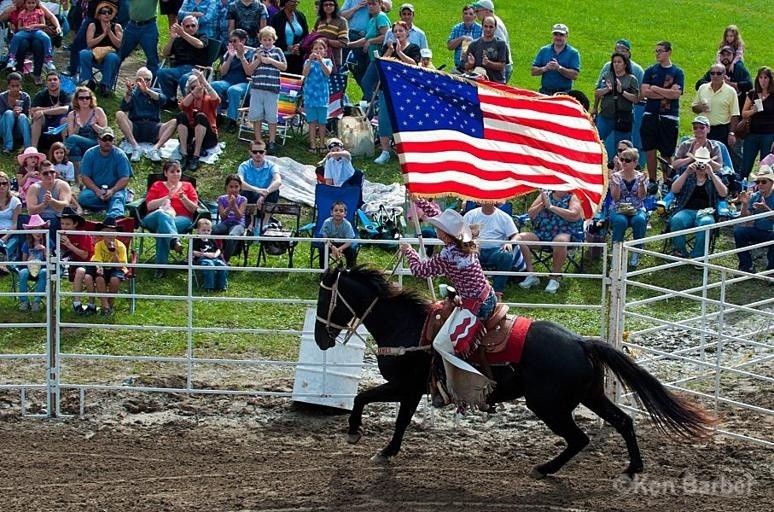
[552,24,568,34]
[473,0,494,12]
[18,146,46,167]
[427,209,472,243]
[95,217,124,232]
[54,207,85,228]
[22,214,50,238]
[420,48,432,59]
[687,147,717,163]
[691,116,710,128]
[99,127,114,138]
[752,165,774,181]
[719,46,736,54]
[400,3,414,12]
[616,40,630,50]
[92,2,118,20]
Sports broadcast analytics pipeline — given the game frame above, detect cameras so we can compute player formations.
[696,162,706,171]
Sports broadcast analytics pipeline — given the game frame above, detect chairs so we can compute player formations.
[256,202,301,268]
[237,72,305,147]
[5,214,59,310]
[297,73,351,150]
[216,204,256,267]
[530,232,585,280]
[125,173,197,270]
[310,166,364,276]
[150,39,222,113]
[68,218,138,313]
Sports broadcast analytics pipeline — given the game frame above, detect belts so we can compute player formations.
[130,17,156,25]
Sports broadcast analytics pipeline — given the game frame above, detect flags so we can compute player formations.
[379,58,609,223]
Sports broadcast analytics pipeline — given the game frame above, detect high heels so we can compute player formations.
[153,270,168,280]
[175,245,185,257]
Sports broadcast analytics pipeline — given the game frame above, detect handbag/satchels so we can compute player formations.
[613,73,633,132]
[735,88,758,140]
[285,14,303,44]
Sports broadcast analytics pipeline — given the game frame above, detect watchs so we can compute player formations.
[728,132,735,136]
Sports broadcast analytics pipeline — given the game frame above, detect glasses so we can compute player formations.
[710,72,724,75]
[41,170,56,176]
[100,10,112,14]
[191,87,195,90]
[323,3,334,7]
[186,24,195,28]
[474,10,485,13]
[47,78,58,81]
[251,150,265,154]
[464,12,473,16]
[754,180,767,184]
[229,39,239,43]
[0,182,8,186]
[693,126,705,130]
[656,49,668,54]
[78,96,90,100]
[618,148,626,152]
[620,158,634,163]
[101,138,113,142]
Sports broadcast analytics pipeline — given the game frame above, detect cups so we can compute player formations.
[289,89,299,101]
[15,98,24,105]
[360,99,367,112]
[755,97,763,112]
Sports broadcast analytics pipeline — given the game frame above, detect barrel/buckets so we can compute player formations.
[291,308,368,411]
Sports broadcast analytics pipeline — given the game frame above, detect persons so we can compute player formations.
[211,174,248,266]
[320,202,357,279]
[238,140,282,234]
[309,1,348,67]
[695,46,752,106]
[348,0,390,119]
[734,164,774,273]
[673,116,723,176]
[339,1,371,81]
[610,149,647,267]
[692,64,739,147]
[227,0,268,34]
[117,66,177,162]
[519,190,585,294]
[741,65,774,179]
[594,54,638,148]
[530,24,580,95]
[303,40,332,154]
[159,16,212,106]
[18,214,54,312]
[1,0,159,91]
[716,26,743,73]
[26,160,72,238]
[211,29,253,132]
[671,146,727,270]
[383,4,429,50]
[270,0,308,75]
[55,207,98,314]
[67,87,107,135]
[595,40,644,148]
[47,142,74,186]
[248,27,287,152]
[0,73,32,155]
[473,0,513,84]
[91,216,128,314]
[16,146,46,206]
[418,49,436,71]
[179,0,219,38]
[459,17,510,79]
[79,126,133,223]
[192,218,228,292]
[462,203,521,303]
[0,172,22,273]
[611,140,632,172]
[27,72,72,149]
[142,160,198,279]
[374,21,421,164]
[447,5,482,71]
[640,41,684,195]
[400,209,497,407]
[324,138,355,187]
[174,69,220,159]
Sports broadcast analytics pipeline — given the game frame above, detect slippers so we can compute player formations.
[319,145,327,155]
[307,147,317,155]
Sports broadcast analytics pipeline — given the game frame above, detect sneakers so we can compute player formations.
[545,279,559,293]
[734,264,756,279]
[179,152,188,171]
[73,303,84,316]
[374,151,391,165]
[43,57,56,70]
[32,76,42,87]
[82,305,97,316]
[101,306,112,317]
[146,148,162,161]
[85,80,96,92]
[689,256,704,271]
[227,120,237,133]
[63,43,73,52]
[18,301,30,313]
[97,81,109,96]
[518,276,540,288]
[664,252,687,268]
[130,147,143,162]
[143,78,150,81]
[7,57,17,68]
[31,301,39,312]
[629,252,640,266]
[269,142,276,154]
[60,71,76,78]
[189,155,200,172]
[3,148,8,155]
[216,115,223,127]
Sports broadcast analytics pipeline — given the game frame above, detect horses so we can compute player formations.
[315,260,717,480]
